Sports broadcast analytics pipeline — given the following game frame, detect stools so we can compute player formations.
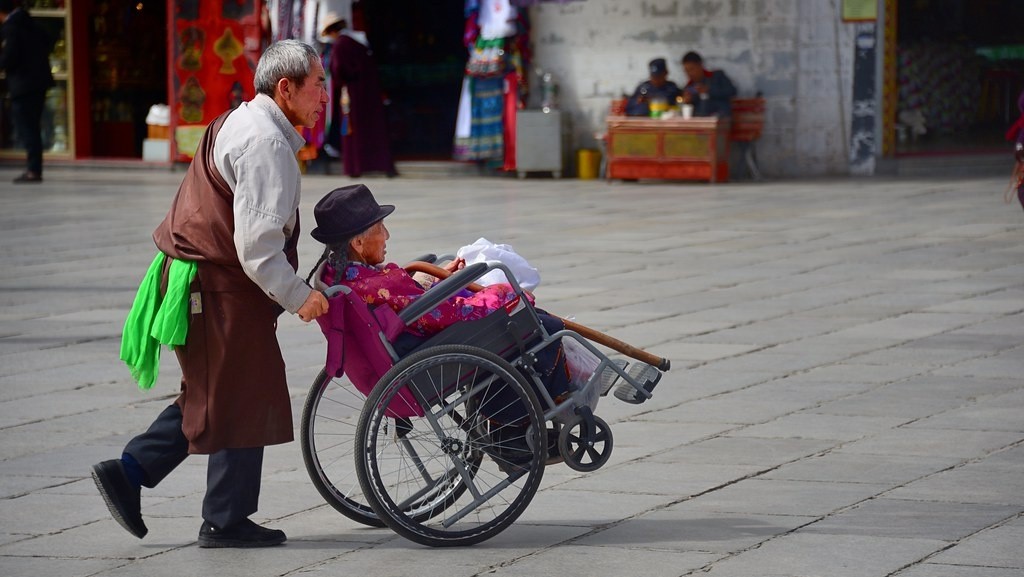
[984,66,1024,128]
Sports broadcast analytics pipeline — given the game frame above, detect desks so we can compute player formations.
[604,116,730,184]
[515,109,573,180]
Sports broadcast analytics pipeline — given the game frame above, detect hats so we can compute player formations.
[310,184,395,244]
[649,59,664,76]
[320,14,346,36]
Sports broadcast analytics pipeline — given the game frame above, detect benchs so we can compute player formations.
[593,95,766,181]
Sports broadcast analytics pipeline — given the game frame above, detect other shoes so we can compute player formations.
[14,173,41,184]
[498,427,572,466]
[90,458,148,540]
[198,518,287,547]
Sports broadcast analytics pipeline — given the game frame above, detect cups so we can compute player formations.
[682,105,694,120]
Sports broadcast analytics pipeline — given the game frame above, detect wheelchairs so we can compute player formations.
[300,252,663,548]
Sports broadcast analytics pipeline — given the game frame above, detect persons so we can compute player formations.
[0,0,55,185]
[622,50,738,118]
[306,183,574,473]
[319,13,398,180]
[89,39,330,548]
